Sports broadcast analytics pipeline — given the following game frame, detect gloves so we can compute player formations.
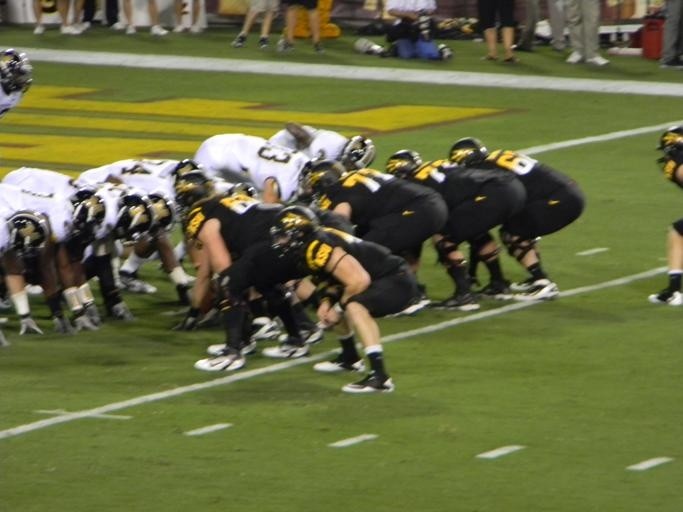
[2,288,132,346]
[174,281,222,331]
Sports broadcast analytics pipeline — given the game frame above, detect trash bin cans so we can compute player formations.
[643,17,665,60]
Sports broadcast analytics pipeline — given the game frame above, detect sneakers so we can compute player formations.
[566,51,583,64]
[313,354,365,373]
[648,288,682,307]
[342,371,393,393]
[586,56,611,66]
[118,269,157,294]
[429,275,557,310]
[382,296,431,320]
[194,316,322,371]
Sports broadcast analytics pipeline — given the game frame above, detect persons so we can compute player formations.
[649,127,683,305]
[2,122,586,391]
[231,0,326,54]
[387,0,438,43]
[478,0,683,65]
[32,1,202,35]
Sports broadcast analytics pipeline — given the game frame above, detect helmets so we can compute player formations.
[7,210,50,258]
[268,135,421,258]
[0,50,33,95]
[66,158,256,241]
[657,126,683,153]
[448,137,488,166]
